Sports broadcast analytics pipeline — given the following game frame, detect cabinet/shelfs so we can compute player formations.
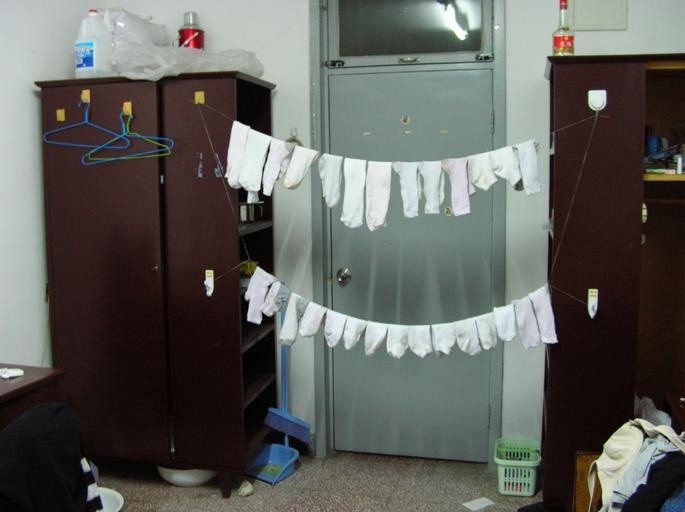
[538,54,684,511]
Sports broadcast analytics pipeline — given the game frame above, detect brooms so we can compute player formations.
[262,278,313,448]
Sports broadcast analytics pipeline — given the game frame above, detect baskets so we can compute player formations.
[492,433,543,498]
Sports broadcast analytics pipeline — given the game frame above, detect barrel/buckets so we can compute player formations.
[72,7,107,78]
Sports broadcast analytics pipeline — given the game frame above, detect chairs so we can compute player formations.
[0,403,87,511]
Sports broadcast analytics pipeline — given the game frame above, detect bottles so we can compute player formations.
[553,0,575,55]
[645,127,683,175]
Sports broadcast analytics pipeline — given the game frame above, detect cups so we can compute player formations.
[240,205,263,224]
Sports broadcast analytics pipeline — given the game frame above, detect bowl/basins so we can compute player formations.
[95,486,123,511]
[157,464,218,487]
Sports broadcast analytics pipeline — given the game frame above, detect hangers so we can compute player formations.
[43,100,173,167]
